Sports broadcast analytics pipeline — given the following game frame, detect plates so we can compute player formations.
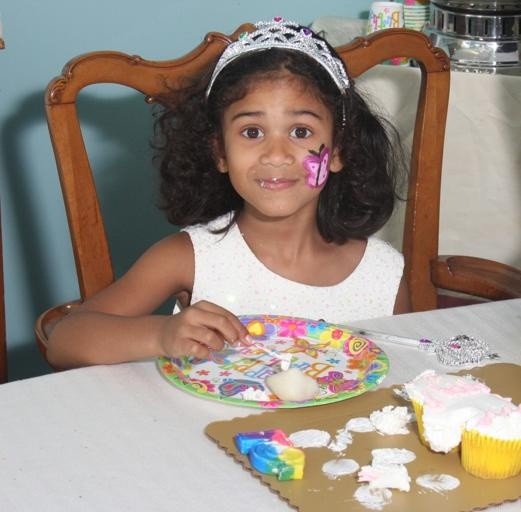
[156,314,389,409]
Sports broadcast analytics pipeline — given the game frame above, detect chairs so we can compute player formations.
[33,22,521,374]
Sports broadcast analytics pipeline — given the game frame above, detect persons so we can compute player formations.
[45,16,411,371]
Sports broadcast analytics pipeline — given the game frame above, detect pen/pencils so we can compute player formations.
[339,324,432,347]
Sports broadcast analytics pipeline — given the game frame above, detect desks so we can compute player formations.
[310,14,521,303]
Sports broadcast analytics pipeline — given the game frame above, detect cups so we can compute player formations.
[396,0,431,32]
[363,3,411,68]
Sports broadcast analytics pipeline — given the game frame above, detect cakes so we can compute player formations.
[406,374,521,479]
